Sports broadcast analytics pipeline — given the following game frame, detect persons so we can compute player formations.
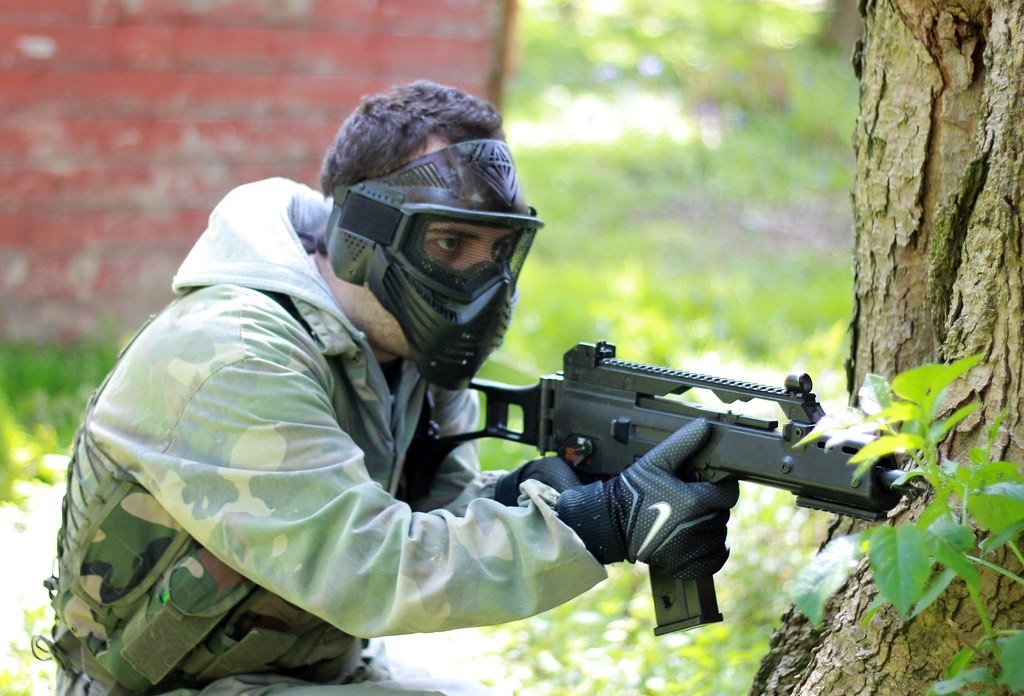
[48,78,742,696]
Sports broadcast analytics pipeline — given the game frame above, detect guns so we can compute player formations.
[398,341,926,639]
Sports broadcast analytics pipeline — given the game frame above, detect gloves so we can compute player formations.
[496,420,739,579]
